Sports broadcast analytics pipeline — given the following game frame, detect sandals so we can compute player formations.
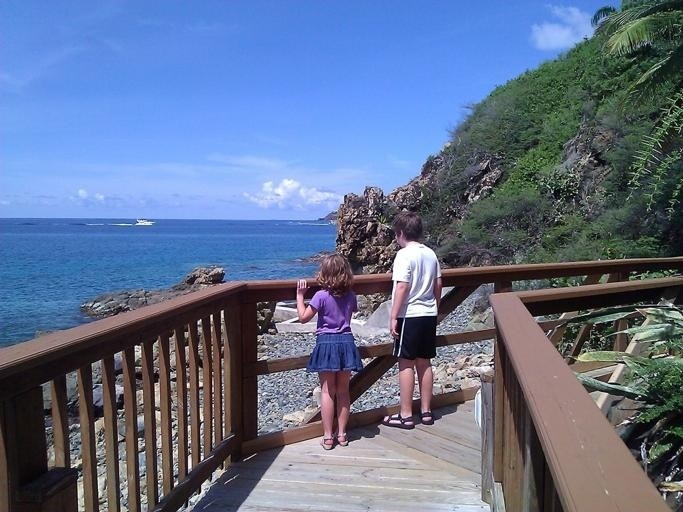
[380,411,415,428]
[319,427,348,450]
[418,408,434,424]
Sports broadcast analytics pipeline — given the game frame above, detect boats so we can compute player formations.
[135,219,154,226]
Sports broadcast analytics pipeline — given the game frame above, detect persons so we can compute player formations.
[296,255,363,451]
[379,211,443,430]
